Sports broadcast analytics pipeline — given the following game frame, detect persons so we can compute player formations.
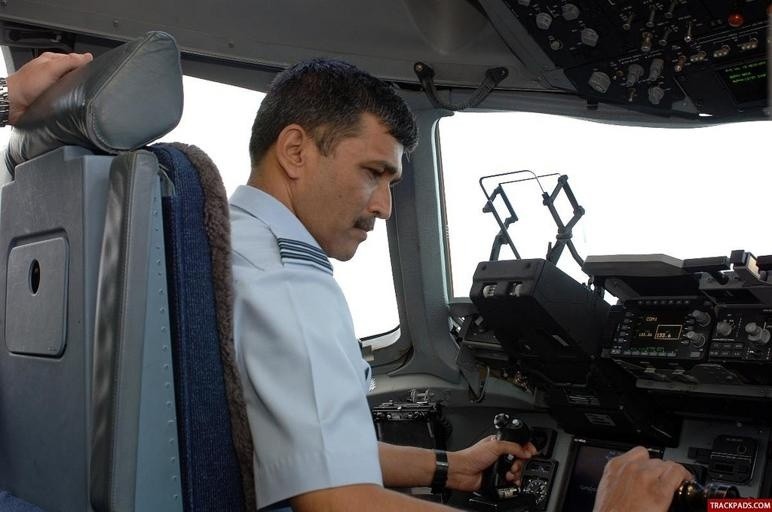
[0,50,94,128]
[224,57,693,512]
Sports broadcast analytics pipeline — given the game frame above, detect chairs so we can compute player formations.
[0,30,256,507]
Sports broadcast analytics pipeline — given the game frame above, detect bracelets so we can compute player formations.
[427,446,450,495]
[0,77,11,128]
